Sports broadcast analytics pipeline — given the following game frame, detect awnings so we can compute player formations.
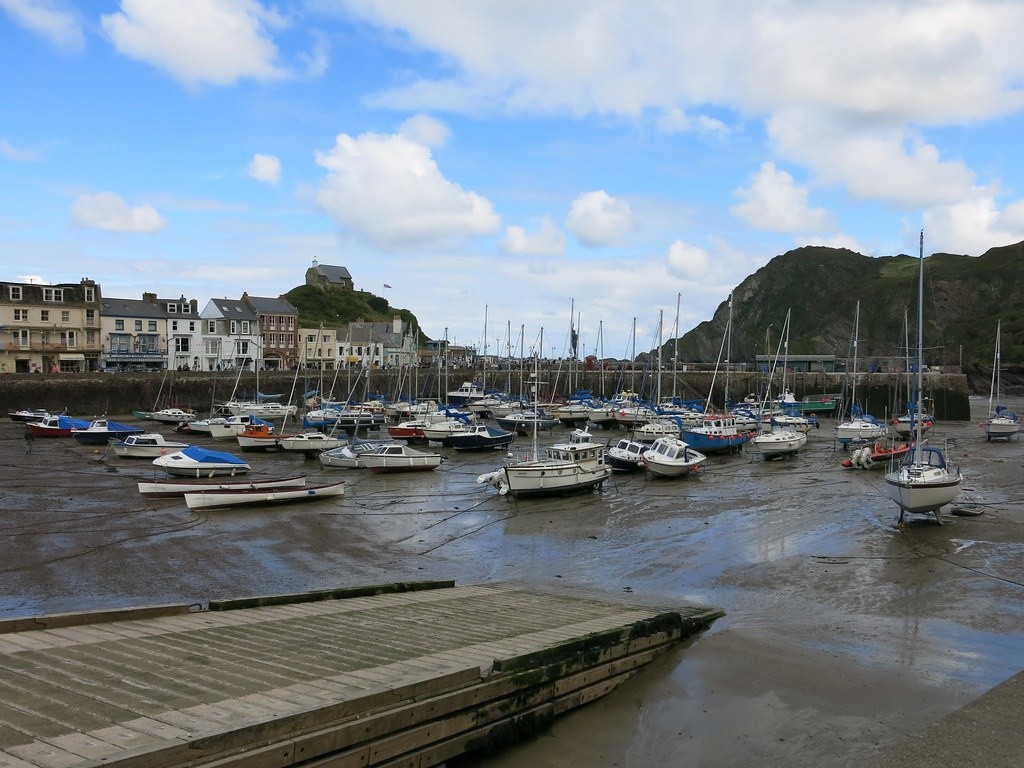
[236,357,253,362]
[58,353,84,361]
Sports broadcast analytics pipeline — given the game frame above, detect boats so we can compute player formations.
[70,415,145,446]
[151,445,251,476]
[182,481,345,513]
[108,434,190,460]
[136,473,309,499]
[8,408,52,424]
[25,411,101,437]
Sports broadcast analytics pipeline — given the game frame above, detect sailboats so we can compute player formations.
[885,229,963,513]
[131,289,935,500]
[982,318,1021,437]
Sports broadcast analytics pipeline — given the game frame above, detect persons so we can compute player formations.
[382,362,391,369]
[178,365,182,371]
[29,363,37,373]
[98,364,104,372]
[25,425,35,457]
[302,363,305,371]
[53,363,60,374]
[209,364,213,371]
[183,363,190,371]
[497,362,503,372]
[217,363,220,371]
[402,359,431,368]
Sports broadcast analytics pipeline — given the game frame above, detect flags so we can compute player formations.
[384,284,391,288]
